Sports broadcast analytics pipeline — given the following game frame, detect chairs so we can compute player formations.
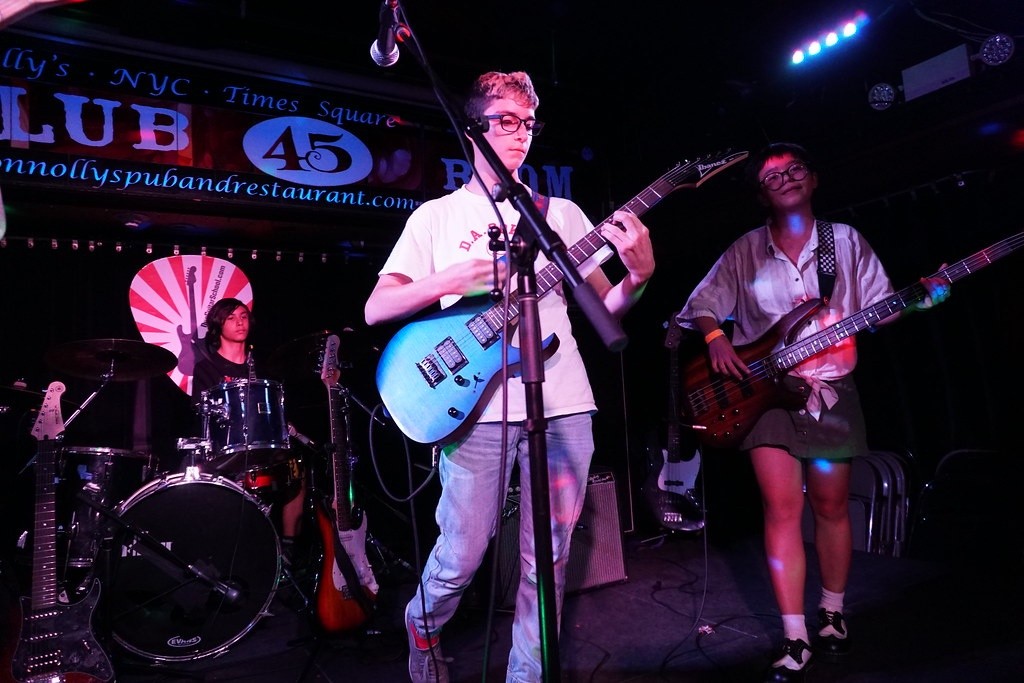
[803,448,1013,572]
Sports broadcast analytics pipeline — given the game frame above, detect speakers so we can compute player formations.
[492,470,628,607]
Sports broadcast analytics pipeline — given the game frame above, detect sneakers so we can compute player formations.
[404,605,454,683]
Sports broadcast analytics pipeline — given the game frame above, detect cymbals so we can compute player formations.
[53,335,180,380]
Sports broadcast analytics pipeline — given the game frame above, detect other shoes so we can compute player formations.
[814,608,848,655]
[282,540,294,567]
[771,638,817,682]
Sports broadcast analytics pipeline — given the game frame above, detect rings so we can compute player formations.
[939,291,948,297]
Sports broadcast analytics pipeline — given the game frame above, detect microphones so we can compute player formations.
[246,346,257,380]
[369,0,400,67]
[225,588,241,607]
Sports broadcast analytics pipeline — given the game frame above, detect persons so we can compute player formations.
[676,142,951,683]
[192,299,307,560]
[365,73,655,683]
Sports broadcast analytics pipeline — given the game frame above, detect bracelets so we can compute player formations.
[705,329,726,345]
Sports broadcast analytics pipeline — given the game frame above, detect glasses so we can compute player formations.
[761,162,812,191]
[483,114,545,136]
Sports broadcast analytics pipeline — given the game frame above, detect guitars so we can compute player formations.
[672,229,1024,451]
[645,319,710,535]
[376,148,748,447]
[311,331,381,634]
[12,381,118,683]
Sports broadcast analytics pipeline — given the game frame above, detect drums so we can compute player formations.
[91,447,285,668]
[203,376,290,461]
[57,443,149,568]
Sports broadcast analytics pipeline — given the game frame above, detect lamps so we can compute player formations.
[868,33,1016,114]
